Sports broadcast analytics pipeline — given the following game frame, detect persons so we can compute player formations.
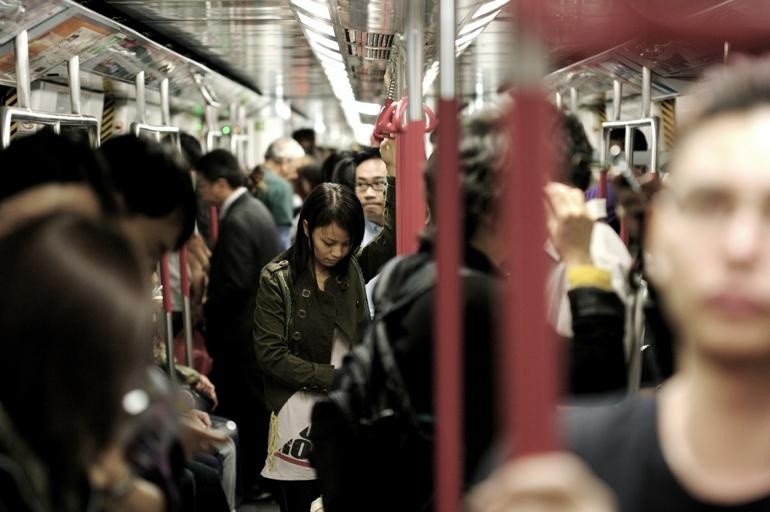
[2,46,770,512]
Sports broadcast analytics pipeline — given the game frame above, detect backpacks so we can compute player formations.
[310,254,479,512]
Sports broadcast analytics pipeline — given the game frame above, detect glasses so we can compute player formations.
[355,181,387,191]
